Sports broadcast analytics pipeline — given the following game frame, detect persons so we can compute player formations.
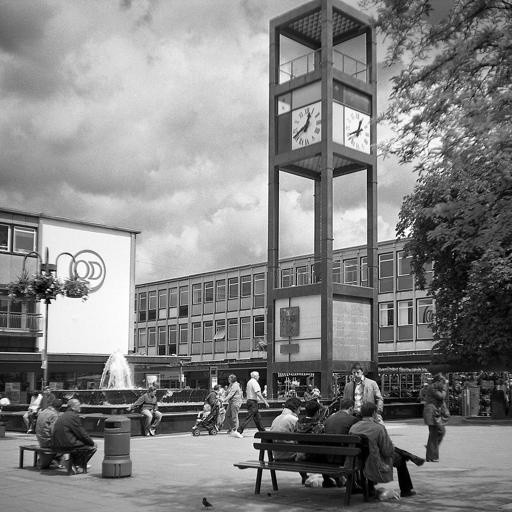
[495,379,507,399]
[0,392,8,412]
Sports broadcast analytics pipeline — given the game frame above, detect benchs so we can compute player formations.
[234,430,378,504]
[18,443,89,476]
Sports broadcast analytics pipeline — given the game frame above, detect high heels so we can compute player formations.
[426,457,439,462]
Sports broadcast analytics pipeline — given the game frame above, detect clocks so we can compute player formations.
[291,99,321,152]
[343,104,372,155]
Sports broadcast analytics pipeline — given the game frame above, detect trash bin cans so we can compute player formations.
[102,415,132,478]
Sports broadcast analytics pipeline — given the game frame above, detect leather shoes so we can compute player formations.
[301,476,363,495]
[400,490,416,497]
[413,456,426,466]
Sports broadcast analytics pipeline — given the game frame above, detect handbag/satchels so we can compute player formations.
[432,404,452,427]
